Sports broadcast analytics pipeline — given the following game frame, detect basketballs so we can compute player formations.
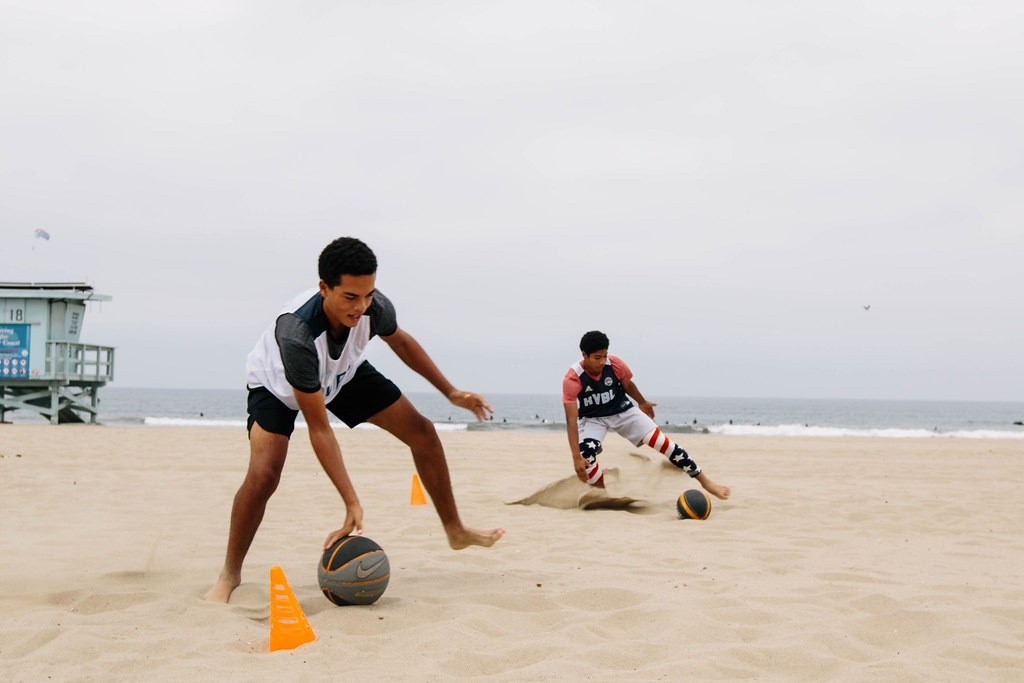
[317,535,391,607]
[675,489,712,520]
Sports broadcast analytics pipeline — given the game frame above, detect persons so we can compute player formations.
[562,331,731,500]
[202,237,506,609]
